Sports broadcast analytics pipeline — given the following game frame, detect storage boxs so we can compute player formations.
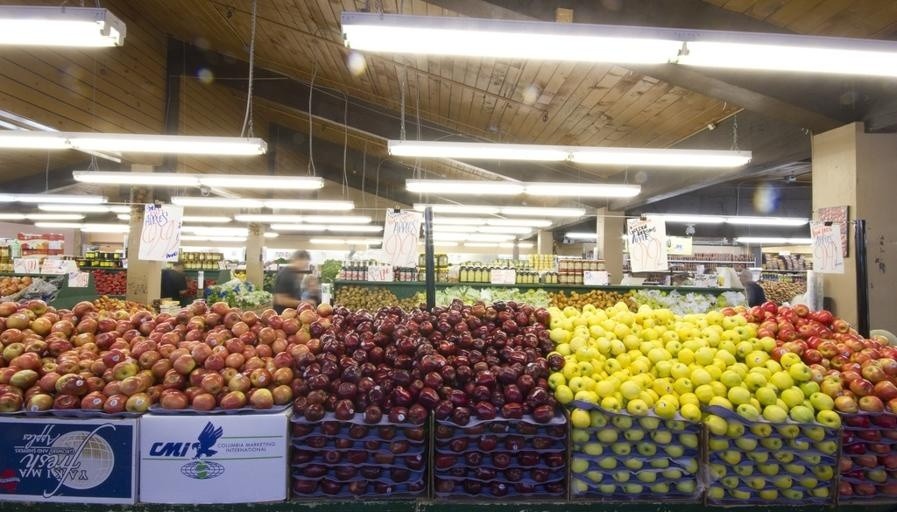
[1,407,290,506]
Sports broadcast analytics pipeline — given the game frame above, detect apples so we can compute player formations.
[534,299,896,504]
[0,298,335,504]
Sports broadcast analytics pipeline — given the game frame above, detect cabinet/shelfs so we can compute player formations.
[617,251,812,285]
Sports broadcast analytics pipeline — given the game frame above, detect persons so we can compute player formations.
[272,250,316,315]
[300,274,321,309]
[161,259,191,307]
[738,270,768,308]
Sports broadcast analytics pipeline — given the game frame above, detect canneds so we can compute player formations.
[64,252,121,267]
[418,253,449,283]
[559,259,606,285]
[183,252,223,271]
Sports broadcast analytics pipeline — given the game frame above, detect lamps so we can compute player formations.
[406,158,641,227]
[389,61,754,169]
[0,0,358,211]
[641,183,807,230]
[338,10,897,78]
